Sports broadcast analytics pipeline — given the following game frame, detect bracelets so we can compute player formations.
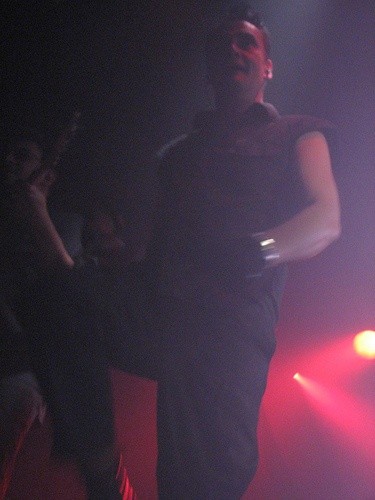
[254,232,281,270]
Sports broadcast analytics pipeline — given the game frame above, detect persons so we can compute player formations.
[0,127,135,500]
[138,8,342,500]
